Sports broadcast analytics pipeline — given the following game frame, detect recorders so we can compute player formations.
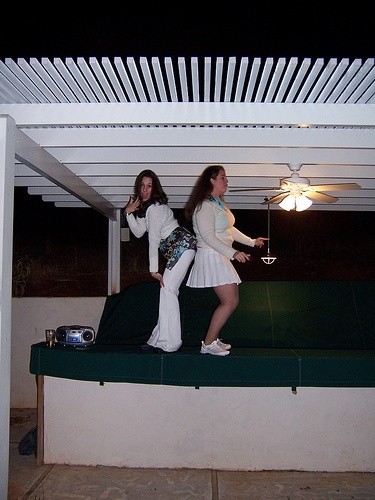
[54,325,95,347]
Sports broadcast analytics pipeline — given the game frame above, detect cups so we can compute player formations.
[45,330,55,349]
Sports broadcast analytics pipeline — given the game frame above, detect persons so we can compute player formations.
[124,168,197,356]
[185,164,271,357]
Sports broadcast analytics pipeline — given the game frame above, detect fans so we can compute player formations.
[228,163,364,206]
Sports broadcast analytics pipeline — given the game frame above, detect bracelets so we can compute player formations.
[124,211,131,216]
[150,272,159,276]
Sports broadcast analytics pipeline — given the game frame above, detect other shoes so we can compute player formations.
[139,344,182,354]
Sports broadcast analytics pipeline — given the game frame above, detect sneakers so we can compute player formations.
[200,338,231,356]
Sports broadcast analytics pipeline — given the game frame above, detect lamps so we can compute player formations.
[278,162,314,212]
